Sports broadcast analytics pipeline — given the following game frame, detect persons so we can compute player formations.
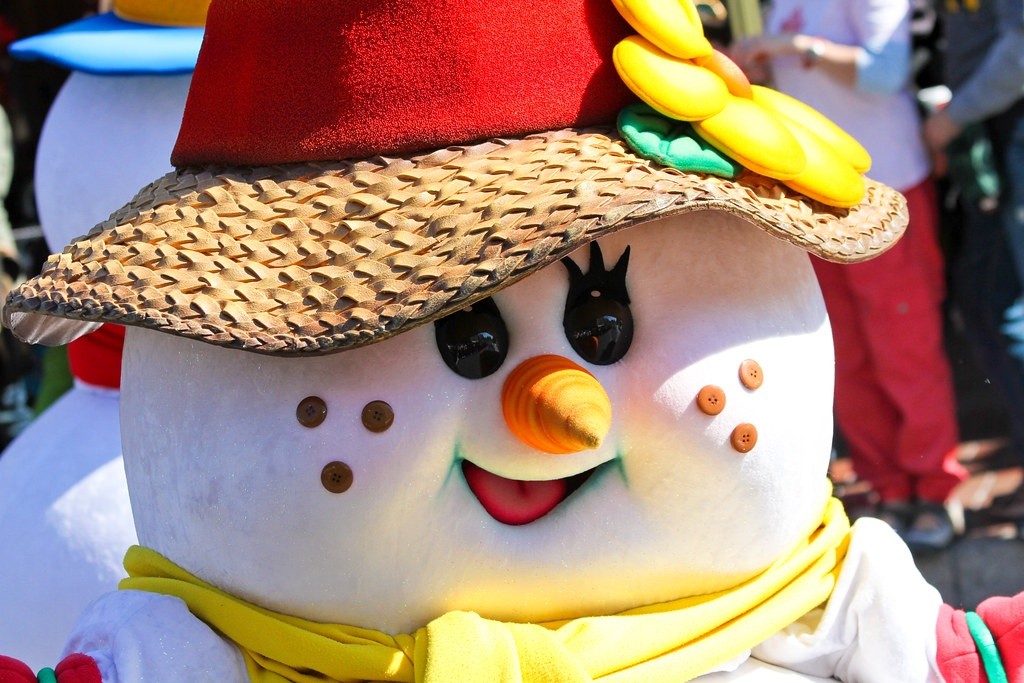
[0,98,51,455]
[924,0,1024,359]
[734,1,967,546]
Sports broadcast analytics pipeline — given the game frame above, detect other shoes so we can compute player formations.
[875,500,953,551]
[981,487,1024,519]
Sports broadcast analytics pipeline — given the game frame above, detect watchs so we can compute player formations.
[803,35,822,71]
